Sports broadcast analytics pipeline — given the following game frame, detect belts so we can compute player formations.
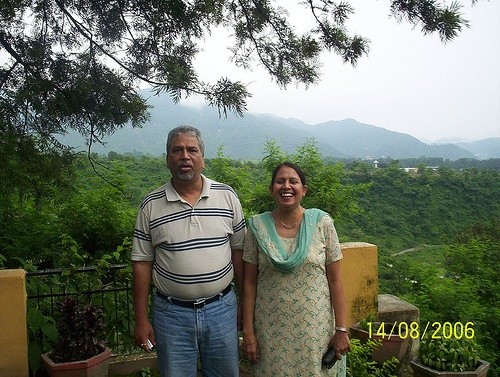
[157,283,232,310]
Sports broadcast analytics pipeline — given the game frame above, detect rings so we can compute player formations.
[140,344,147,347]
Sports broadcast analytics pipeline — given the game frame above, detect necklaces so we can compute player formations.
[277,212,302,229]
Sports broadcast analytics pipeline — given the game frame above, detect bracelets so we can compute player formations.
[244,338,257,345]
[336,326,348,332]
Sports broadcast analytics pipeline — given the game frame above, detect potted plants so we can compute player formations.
[410,326,490,377]
[350,315,409,365]
[42,294,110,377]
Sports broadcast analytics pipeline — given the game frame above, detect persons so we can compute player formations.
[242,163,352,377]
[131,125,248,377]
